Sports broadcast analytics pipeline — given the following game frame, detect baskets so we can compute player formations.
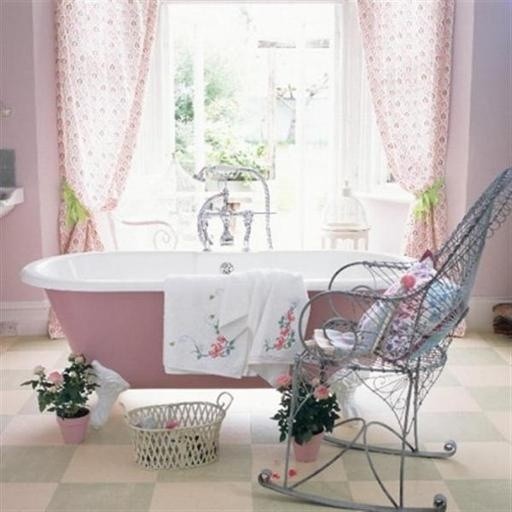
[117,391,234,472]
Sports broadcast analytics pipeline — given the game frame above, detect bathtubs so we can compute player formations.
[18,251,455,391]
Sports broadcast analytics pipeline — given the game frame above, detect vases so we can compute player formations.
[288,430,322,462]
[56,411,89,447]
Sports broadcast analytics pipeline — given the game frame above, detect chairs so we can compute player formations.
[258,164,512,512]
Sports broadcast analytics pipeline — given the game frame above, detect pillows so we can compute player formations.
[350,248,472,366]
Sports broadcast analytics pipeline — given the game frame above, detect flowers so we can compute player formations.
[270,370,343,444]
[17,349,104,417]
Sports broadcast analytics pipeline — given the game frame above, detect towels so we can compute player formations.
[215,262,312,391]
[159,267,248,384]
